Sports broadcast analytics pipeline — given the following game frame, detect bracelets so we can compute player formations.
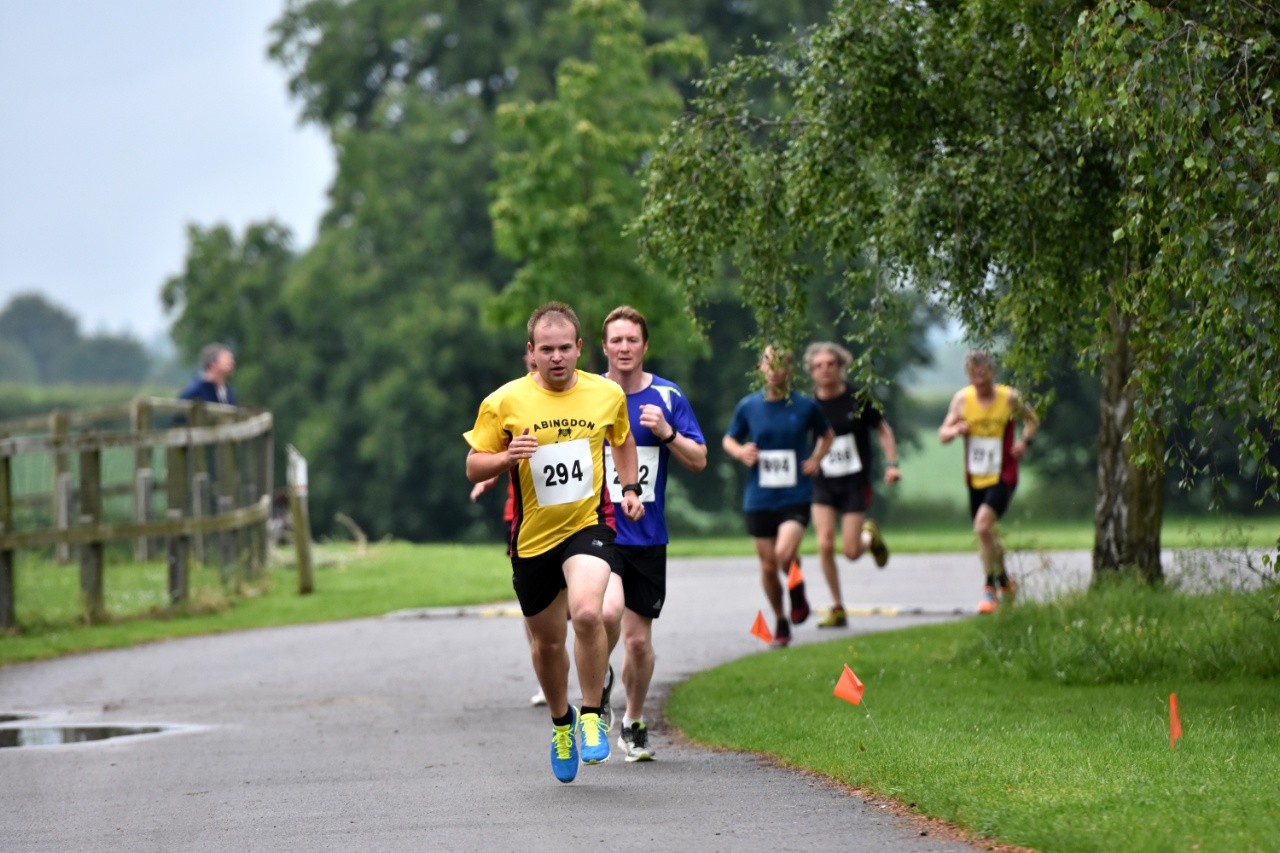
[1021,437,1033,445]
[883,461,900,467]
[659,427,675,444]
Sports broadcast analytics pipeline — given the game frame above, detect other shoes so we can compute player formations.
[531,688,545,704]
[776,617,791,644]
[790,600,809,623]
[862,519,888,567]
[978,585,996,613]
[997,580,1019,610]
[817,606,847,627]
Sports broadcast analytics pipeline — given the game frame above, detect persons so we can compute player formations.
[458,302,645,784]
[172,343,238,454]
[597,303,707,763]
[722,345,834,647]
[939,353,1038,615]
[802,340,899,629]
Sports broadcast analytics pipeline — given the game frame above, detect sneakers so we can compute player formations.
[617,722,657,763]
[599,665,616,733]
[579,713,611,765]
[550,705,580,783]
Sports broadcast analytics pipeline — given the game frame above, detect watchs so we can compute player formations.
[621,482,642,498]
